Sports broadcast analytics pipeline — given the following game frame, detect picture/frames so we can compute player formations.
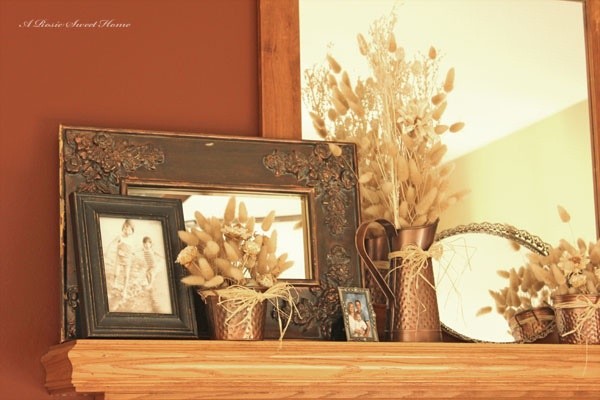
[57,125,365,345]
[70,192,199,341]
[338,286,380,341]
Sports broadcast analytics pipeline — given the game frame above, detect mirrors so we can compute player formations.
[433,222,566,343]
[120,177,321,287]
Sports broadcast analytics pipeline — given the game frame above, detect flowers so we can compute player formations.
[170,195,301,305]
[302,9,470,230]
[475,207,599,315]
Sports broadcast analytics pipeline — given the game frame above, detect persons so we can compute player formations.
[104,218,135,297]
[355,300,371,337]
[354,311,367,337]
[133,236,166,290]
[347,302,355,337]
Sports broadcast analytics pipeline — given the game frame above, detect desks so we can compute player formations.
[70,341,600,400]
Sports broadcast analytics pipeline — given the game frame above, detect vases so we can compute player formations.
[201,292,268,342]
[508,307,558,344]
[552,293,600,345]
